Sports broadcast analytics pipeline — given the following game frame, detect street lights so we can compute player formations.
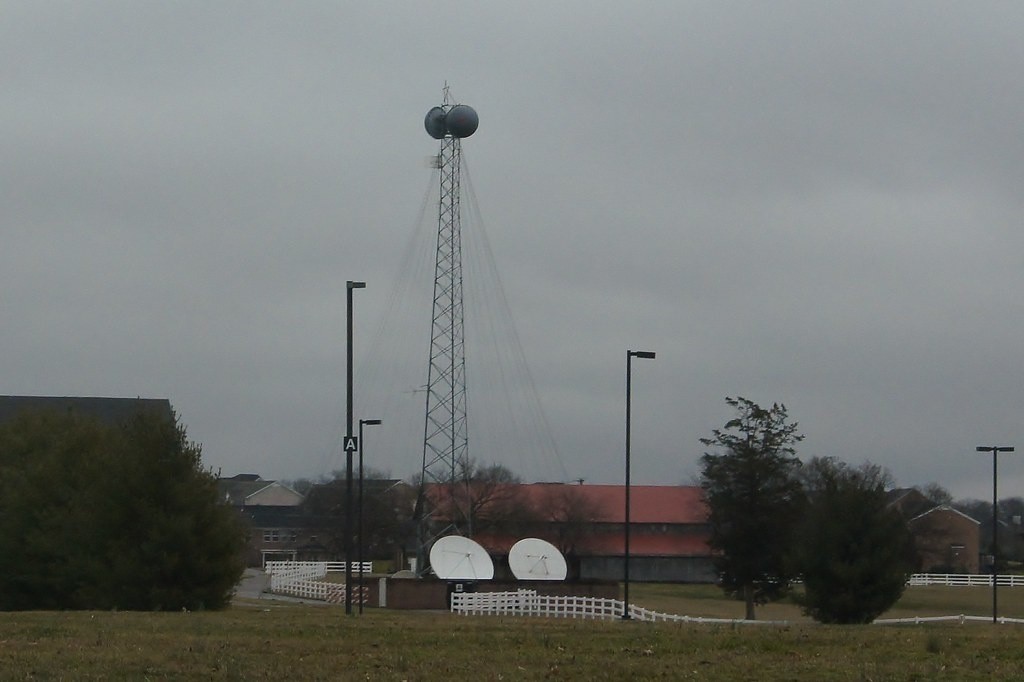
[343,278,368,616]
[976,445,1016,624]
[359,419,382,614]
[621,349,656,619]
